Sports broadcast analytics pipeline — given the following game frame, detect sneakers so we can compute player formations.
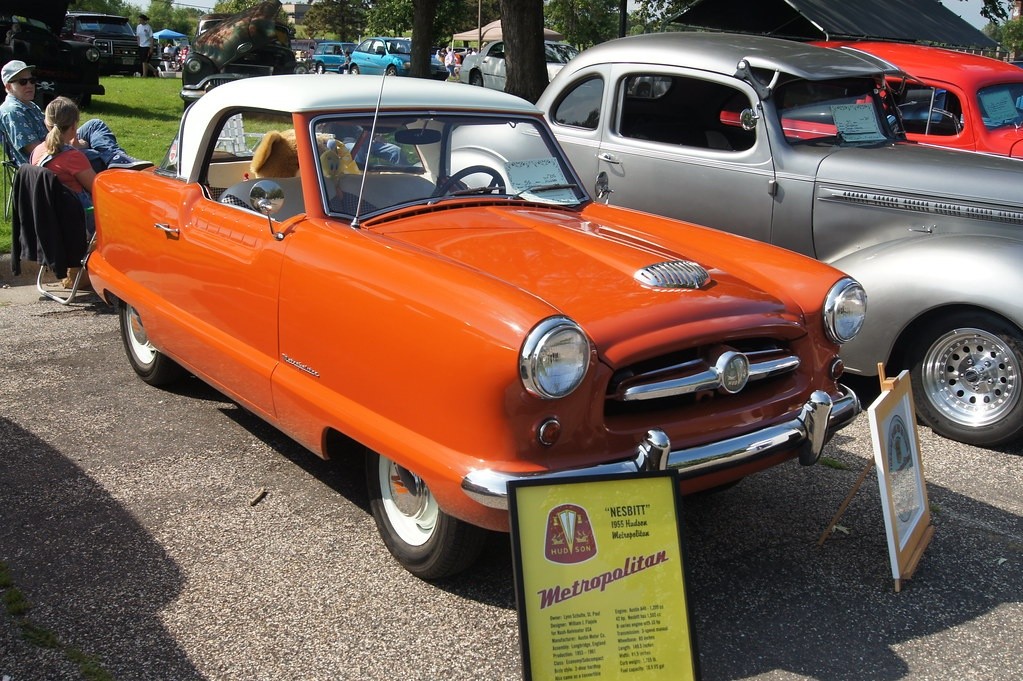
[108,152,154,171]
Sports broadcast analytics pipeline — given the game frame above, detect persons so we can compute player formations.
[471,47,477,55]
[435,49,445,60]
[445,46,458,83]
[173,43,182,68]
[466,50,471,57]
[185,45,190,55]
[338,52,351,74]
[163,44,172,71]
[309,46,315,59]
[136,14,159,78]
[436,50,444,63]
[451,20,564,52]
[29,96,97,242]
[481,42,487,52]
[181,49,186,69]
[322,122,411,167]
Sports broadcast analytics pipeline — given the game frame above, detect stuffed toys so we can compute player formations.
[252,129,352,178]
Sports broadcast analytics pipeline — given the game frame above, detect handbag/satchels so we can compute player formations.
[61,266,90,288]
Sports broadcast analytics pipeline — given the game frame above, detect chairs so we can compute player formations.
[11,165,97,305]
[0,130,20,222]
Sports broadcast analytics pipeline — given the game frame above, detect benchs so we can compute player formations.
[214,174,451,222]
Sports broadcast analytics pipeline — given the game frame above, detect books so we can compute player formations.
[0,60,154,171]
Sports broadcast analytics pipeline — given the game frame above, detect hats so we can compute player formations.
[1,60,36,85]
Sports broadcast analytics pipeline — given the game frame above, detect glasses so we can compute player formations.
[7,76,35,86]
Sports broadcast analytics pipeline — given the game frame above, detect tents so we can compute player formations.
[153,29,187,46]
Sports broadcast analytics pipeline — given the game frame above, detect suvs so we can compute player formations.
[312,42,360,75]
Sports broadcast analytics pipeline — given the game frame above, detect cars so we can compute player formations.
[531,32,1023,447]
[721,40,1023,164]
[347,37,450,81]
[178,0,296,112]
[87,71,867,586]
[458,39,583,101]
[59,11,139,75]
